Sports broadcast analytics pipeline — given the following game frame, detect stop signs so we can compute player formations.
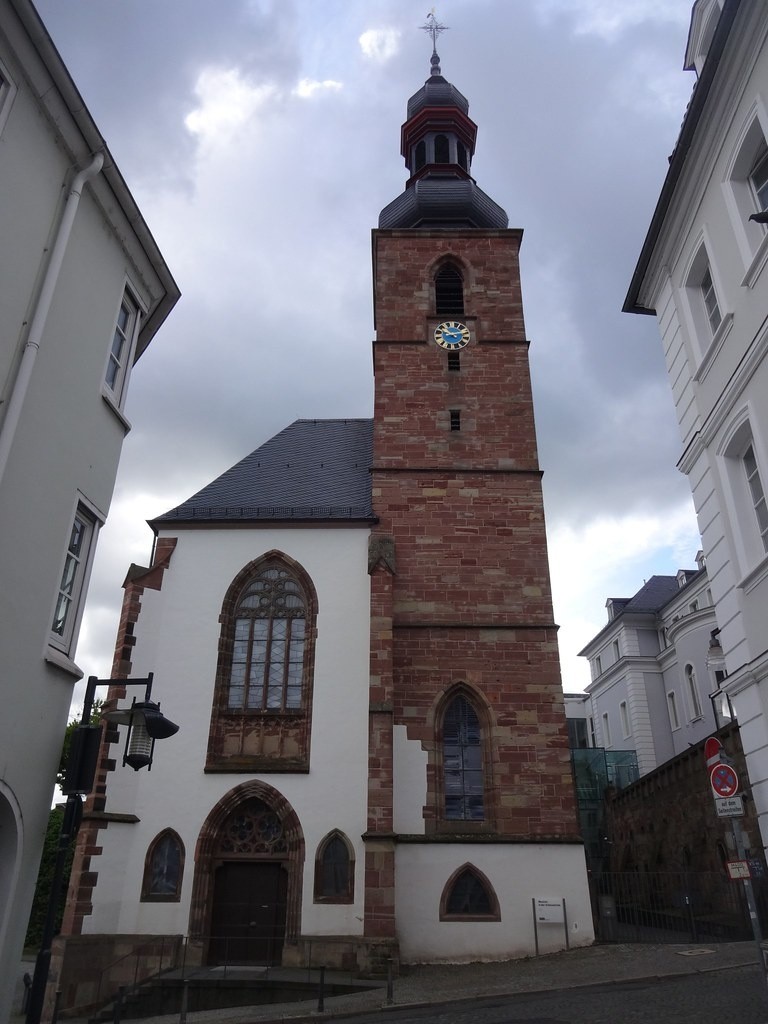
[705,737,723,773]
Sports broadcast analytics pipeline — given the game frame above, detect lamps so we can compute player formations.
[705,627,727,671]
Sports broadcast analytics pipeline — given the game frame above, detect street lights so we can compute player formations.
[26,670,180,1024]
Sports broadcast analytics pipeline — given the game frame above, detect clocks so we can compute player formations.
[433,321,471,350]
